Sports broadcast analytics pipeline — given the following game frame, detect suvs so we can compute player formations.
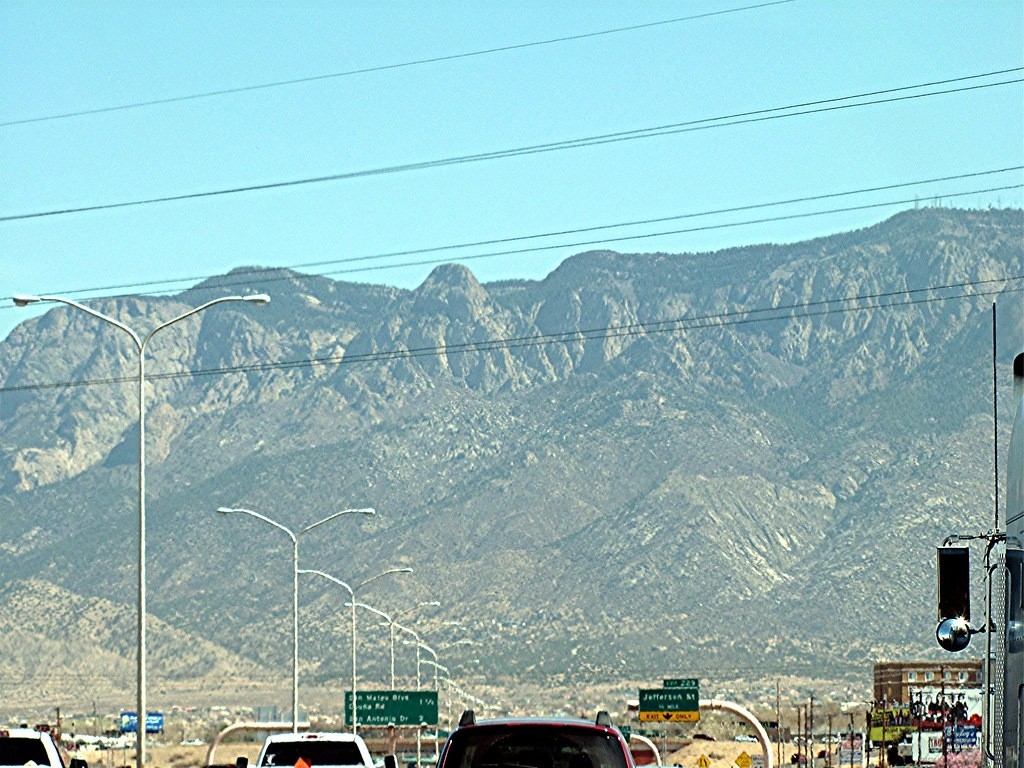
[0,726,89,768]
[436,708,638,768]
[236,732,398,768]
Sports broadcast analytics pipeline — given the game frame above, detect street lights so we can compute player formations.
[296,568,415,732]
[216,506,377,733]
[344,601,439,691]
[12,294,272,768]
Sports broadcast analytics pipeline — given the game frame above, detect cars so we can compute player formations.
[693,733,717,742]
[733,734,759,744]
[795,735,840,746]
